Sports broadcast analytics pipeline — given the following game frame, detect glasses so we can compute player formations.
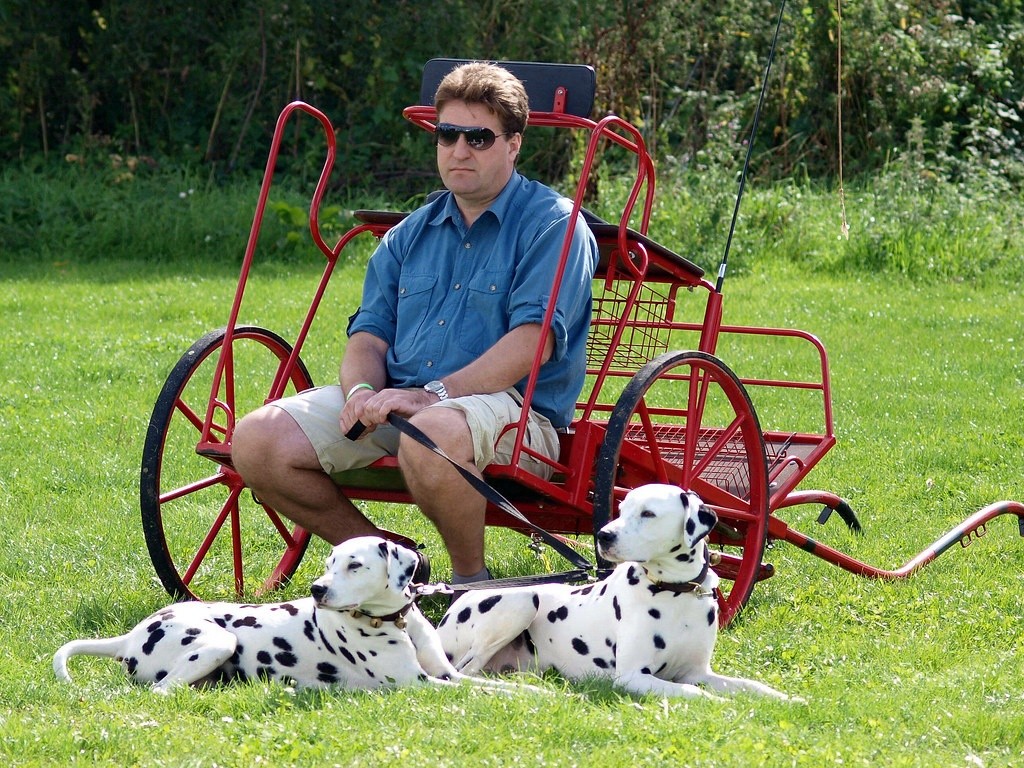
[435,123,508,150]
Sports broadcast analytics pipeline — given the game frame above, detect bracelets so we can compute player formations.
[346,384,373,401]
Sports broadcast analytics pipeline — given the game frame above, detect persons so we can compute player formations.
[232,61,600,607]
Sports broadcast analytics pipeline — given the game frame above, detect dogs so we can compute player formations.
[435,482,809,710]
[48,534,588,707]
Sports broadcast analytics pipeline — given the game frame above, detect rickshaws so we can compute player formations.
[137,58,1024,641]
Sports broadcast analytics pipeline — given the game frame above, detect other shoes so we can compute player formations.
[412,551,431,602]
[449,568,494,607]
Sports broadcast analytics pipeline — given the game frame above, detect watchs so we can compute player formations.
[424,381,448,401]
